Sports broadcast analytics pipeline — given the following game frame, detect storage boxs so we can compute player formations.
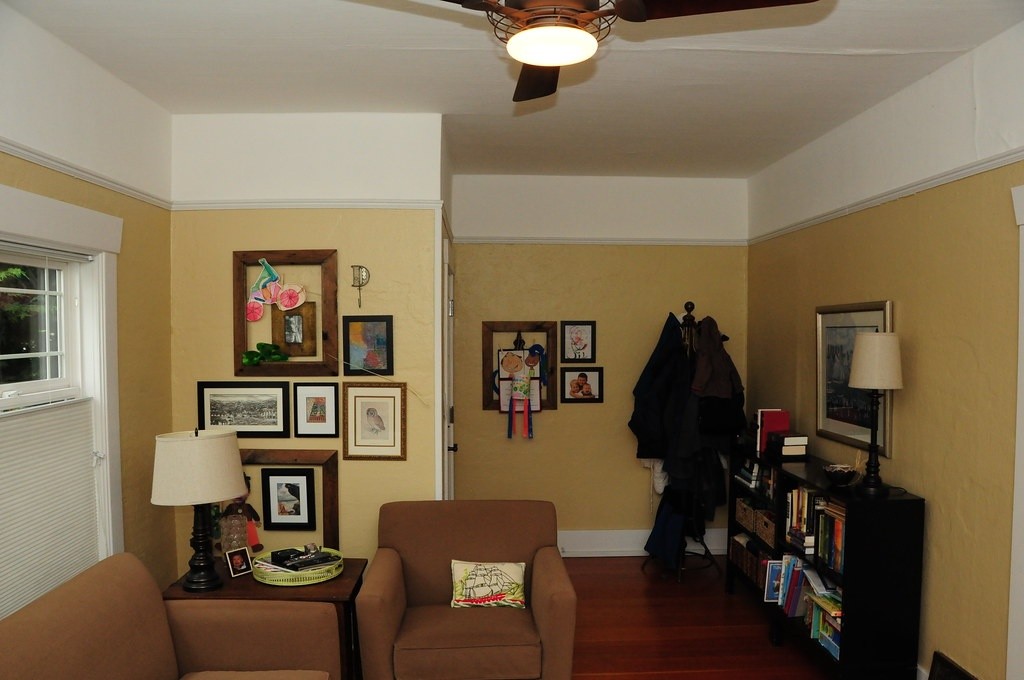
[734,498,777,549]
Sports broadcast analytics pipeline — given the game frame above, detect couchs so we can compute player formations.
[355,499,577,680]
[0,552,341,680]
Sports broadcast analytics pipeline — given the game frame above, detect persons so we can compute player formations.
[232,554,249,574]
[210,399,277,425]
[569,372,594,398]
[215,472,263,553]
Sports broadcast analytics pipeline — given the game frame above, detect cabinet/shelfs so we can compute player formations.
[722,448,925,680]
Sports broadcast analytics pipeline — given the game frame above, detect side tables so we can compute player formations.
[164,553,370,680]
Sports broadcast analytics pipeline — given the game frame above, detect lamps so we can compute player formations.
[847,332,904,501]
[150,429,249,593]
[484,0,615,67]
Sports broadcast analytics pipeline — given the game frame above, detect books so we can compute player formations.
[736,407,844,661]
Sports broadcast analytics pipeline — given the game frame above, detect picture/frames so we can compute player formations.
[207,449,339,550]
[224,547,253,578]
[293,382,339,439]
[561,321,596,363]
[342,315,407,460]
[232,248,340,377]
[816,300,892,459]
[560,367,604,403]
[197,381,290,438]
[481,320,557,410]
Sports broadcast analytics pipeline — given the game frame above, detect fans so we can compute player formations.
[441,0,820,101]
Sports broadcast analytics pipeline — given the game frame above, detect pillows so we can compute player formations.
[449,556,527,608]
